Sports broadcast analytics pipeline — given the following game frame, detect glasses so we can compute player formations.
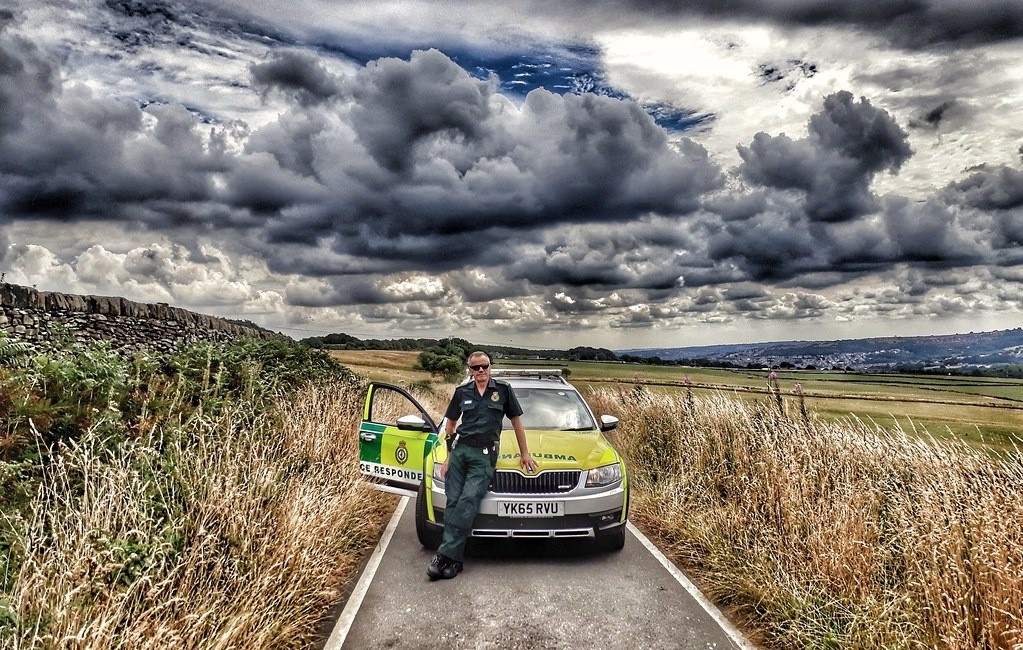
[469,364,489,371]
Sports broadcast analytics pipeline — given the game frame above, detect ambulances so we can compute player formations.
[357,368,629,549]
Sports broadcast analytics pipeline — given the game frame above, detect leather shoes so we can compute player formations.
[426,555,448,578]
[440,561,463,579]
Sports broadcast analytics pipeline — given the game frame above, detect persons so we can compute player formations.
[426,351,539,580]
